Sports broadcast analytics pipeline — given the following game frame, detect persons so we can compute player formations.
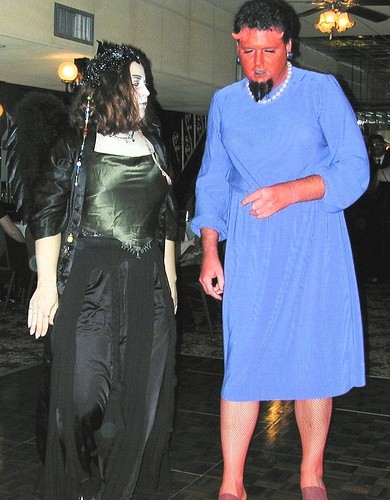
[27,43,178,500]
[189,0,369,500]
[369,135,390,281]
[0,204,26,244]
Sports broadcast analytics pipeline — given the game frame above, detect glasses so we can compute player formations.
[372,142,383,146]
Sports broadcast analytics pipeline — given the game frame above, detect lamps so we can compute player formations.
[57,62,78,92]
[314,4,355,34]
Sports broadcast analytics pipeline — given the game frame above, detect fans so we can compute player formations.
[282,0,390,23]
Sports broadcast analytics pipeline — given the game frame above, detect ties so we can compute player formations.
[377,159,381,165]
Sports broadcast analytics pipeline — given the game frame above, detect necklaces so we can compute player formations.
[108,131,135,142]
[248,61,292,103]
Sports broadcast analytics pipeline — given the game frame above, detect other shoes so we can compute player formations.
[73,452,92,483]
[301,483,327,500]
[219,486,247,500]
